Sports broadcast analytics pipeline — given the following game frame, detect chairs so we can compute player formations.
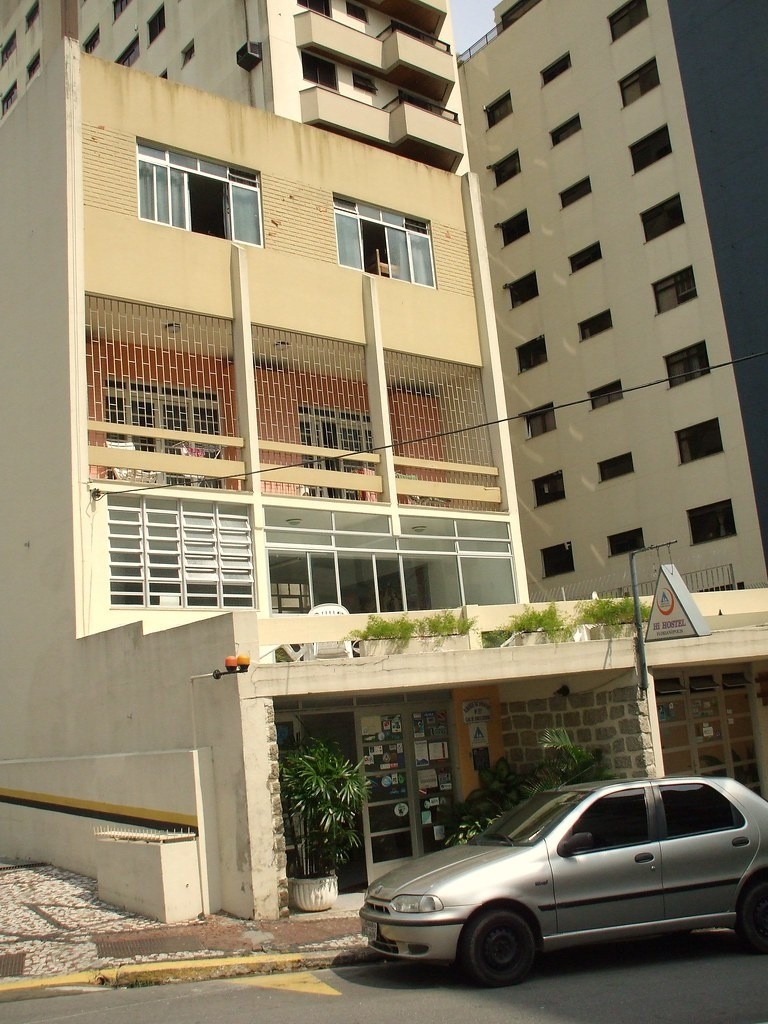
[105,441,160,484]
[302,602,354,661]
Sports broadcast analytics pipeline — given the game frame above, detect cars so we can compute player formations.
[357,775,768,990]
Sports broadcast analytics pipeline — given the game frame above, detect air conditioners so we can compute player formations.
[234,40,262,71]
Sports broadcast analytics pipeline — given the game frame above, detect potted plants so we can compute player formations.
[481,591,653,646]
[279,733,375,911]
[339,605,481,658]
